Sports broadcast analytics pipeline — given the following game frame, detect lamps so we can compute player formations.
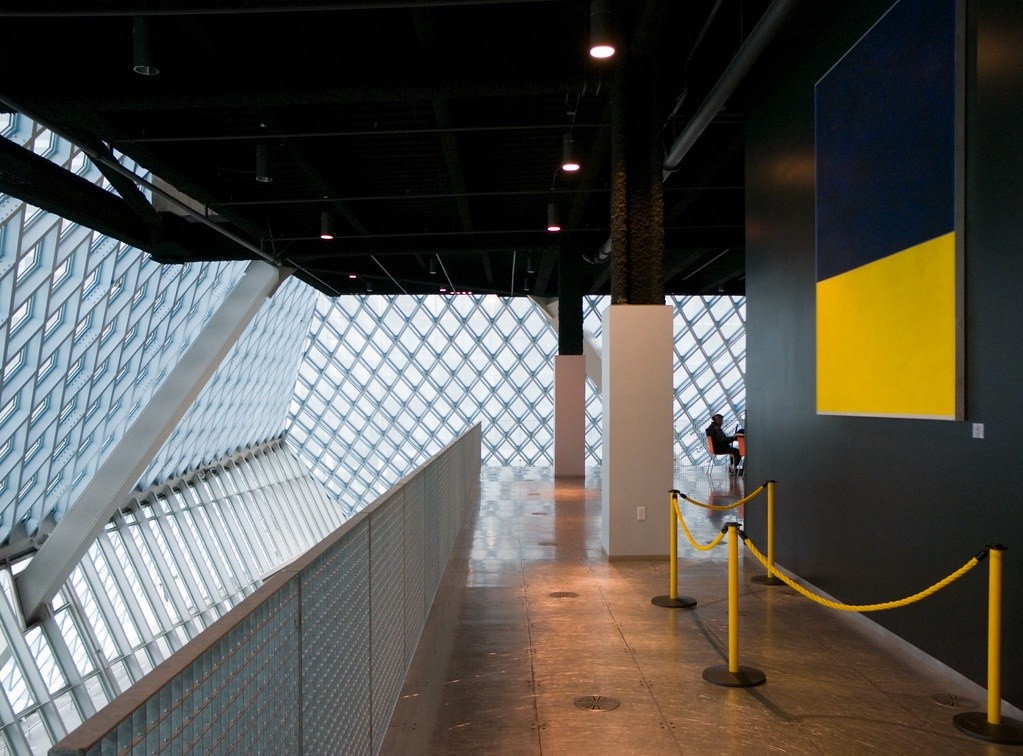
[546,202,562,231]
[429,259,436,274]
[562,133,581,171]
[320,212,334,240]
[589,0,615,58]
[256,145,274,183]
[133,15,161,74]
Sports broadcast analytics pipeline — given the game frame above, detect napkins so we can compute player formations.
[528,258,534,274]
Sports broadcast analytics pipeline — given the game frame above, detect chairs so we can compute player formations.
[737,437,745,476]
[706,435,736,476]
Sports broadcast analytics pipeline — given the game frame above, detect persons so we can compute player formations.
[706,414,741,474]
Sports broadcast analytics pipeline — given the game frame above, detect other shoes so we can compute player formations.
[729,467,739,473]
